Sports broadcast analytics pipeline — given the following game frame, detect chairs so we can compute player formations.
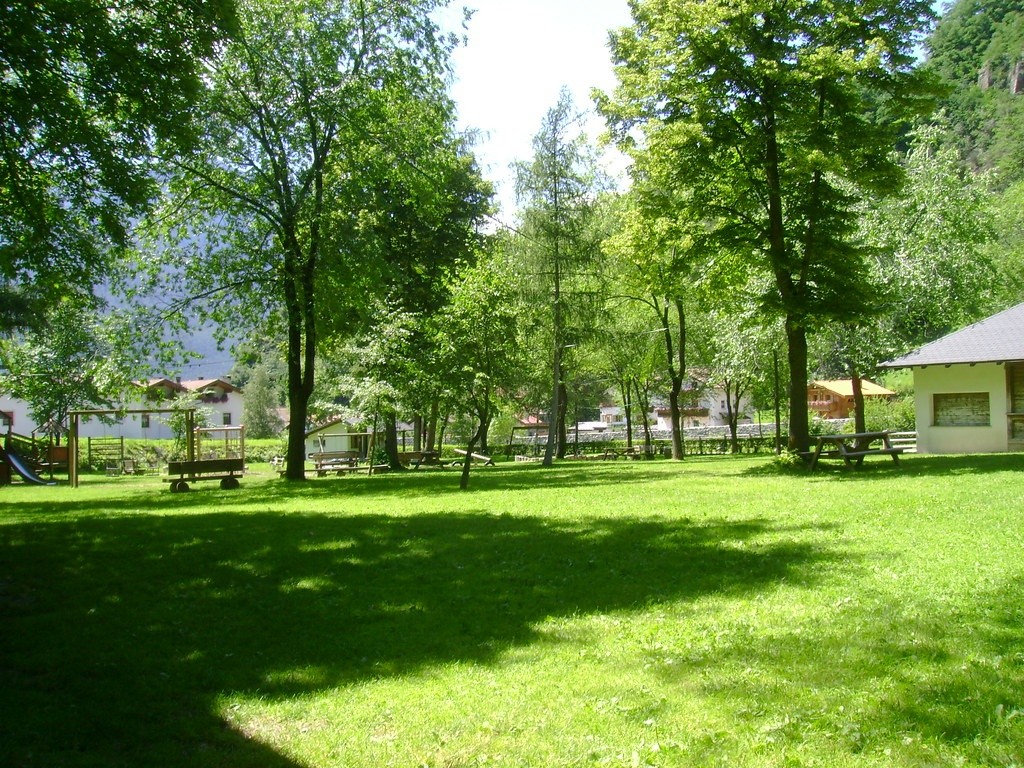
[124,460,136,475]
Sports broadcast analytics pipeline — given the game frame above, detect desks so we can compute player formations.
[603,448,634,461]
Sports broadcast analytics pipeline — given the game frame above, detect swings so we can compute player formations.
[102,413,121,477]
[142,411,161,477]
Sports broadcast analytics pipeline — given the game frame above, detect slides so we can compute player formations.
[4,447,53,484]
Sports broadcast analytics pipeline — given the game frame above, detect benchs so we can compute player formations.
[796,450,842,471]
[840,448,904,469]
[626,453,646,460]
[598,453,618,461]
[808,430,902,473]
